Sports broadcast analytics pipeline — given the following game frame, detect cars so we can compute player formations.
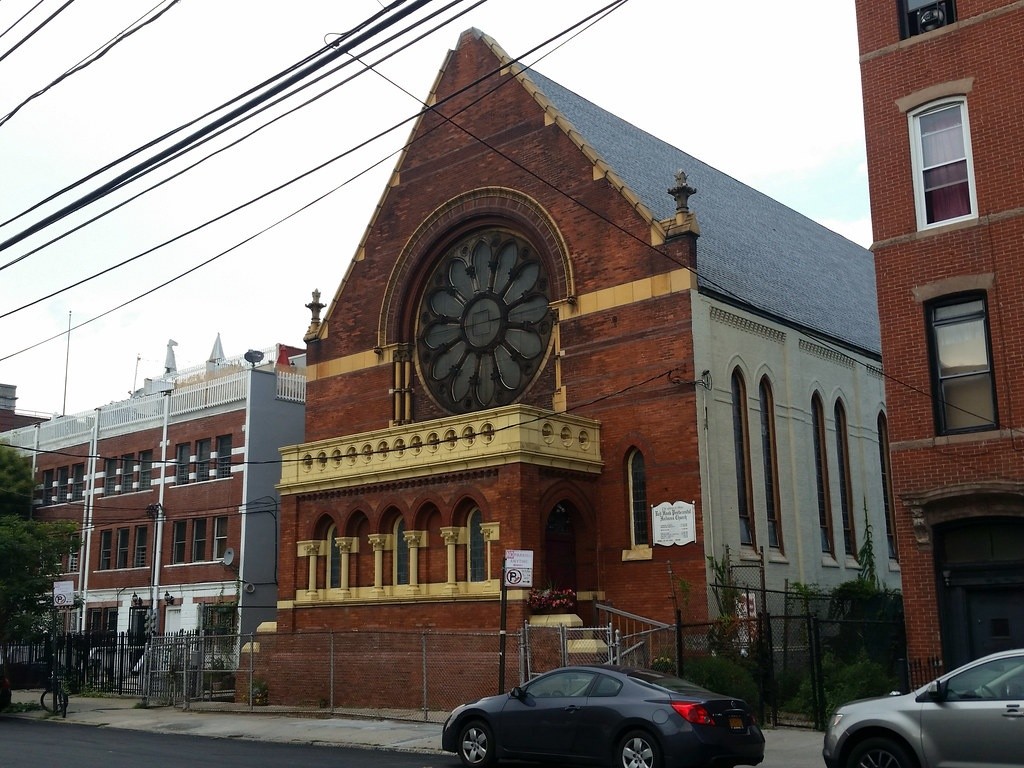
[823,648,1024,768]
[443,662,768,768]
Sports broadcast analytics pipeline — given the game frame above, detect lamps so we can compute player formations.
[164,590,174,606]
[132,591,142,606]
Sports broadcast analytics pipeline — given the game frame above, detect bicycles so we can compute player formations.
[39,673,70,719]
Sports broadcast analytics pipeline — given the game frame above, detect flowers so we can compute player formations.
[526,587,576,610]
